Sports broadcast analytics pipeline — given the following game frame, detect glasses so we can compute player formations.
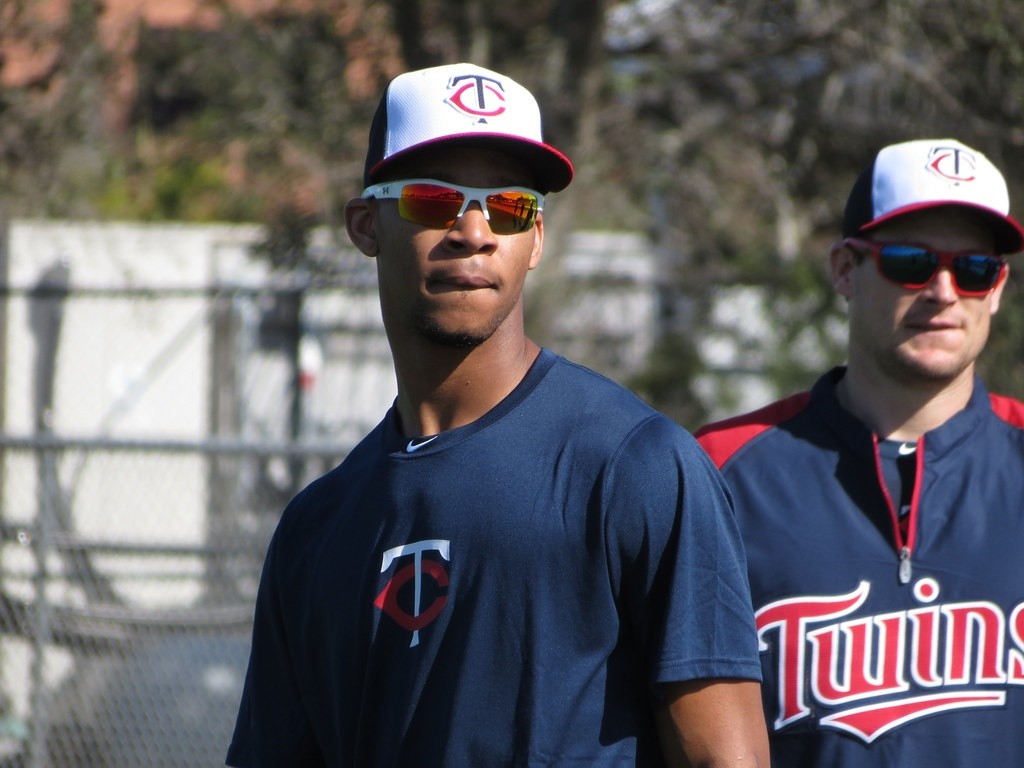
[359,178,546,235]
[843,236,1011,298]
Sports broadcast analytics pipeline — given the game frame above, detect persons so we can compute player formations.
[222,60,771,767]
[687,137,1024,767]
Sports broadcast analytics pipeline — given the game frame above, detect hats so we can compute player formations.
[841,139,1023,257]
[361,61,574,193]
